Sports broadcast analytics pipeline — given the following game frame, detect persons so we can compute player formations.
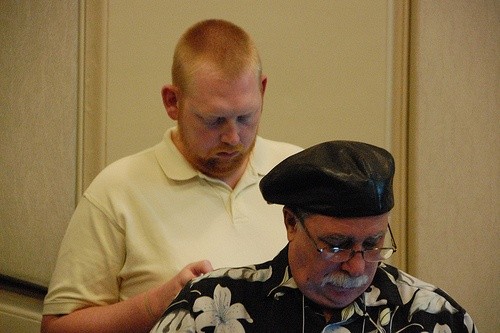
[41,17,306,333]
[151,141,476,333]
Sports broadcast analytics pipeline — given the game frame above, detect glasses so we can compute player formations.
[295,213,398,264]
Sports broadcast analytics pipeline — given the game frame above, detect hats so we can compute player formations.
[258,139,395,216]
